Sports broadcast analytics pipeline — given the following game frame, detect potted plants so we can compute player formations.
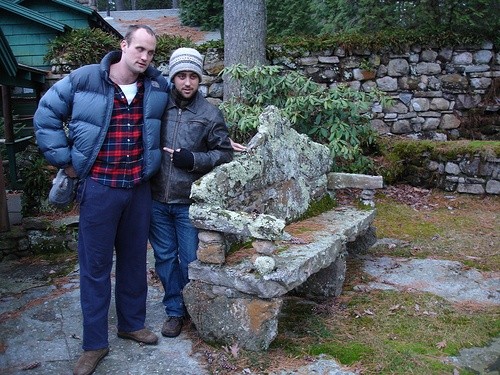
[43,28,118,88]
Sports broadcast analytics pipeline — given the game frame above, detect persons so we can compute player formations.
[33,24,248,375]
[149,48,233,336]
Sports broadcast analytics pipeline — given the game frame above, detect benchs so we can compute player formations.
[183,105,384,351]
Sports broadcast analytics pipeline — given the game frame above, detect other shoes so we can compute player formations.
[116,328,159,343]
[161,315,182,338]
[74,346,109,375]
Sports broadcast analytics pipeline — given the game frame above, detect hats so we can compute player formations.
[169,48,203,82]
